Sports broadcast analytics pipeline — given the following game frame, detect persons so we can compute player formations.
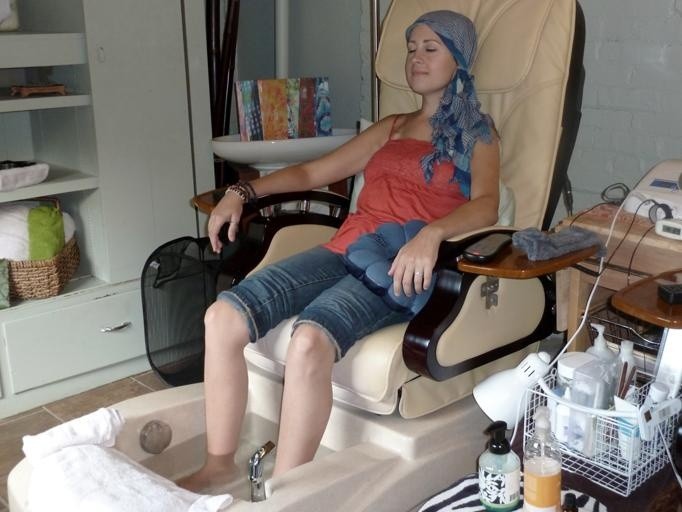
[176,9,497,495]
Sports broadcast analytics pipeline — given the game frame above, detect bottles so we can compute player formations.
[616,338,637,385]
[556,351,596,397]
[643,383,670,409]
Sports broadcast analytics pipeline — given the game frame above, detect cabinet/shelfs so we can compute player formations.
[458,202,682,352]
[0,0,216,420]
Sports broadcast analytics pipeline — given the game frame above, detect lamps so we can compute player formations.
[472,350,668,442]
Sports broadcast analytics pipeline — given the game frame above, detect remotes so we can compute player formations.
[462,232,513,265]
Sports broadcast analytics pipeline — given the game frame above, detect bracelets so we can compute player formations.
[224,180,256,203]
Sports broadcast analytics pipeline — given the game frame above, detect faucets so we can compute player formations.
[247,439,277,502]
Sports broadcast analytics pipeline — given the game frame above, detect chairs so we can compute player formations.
[8,1,586,512]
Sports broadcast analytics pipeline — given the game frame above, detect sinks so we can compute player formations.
[106,381,366,512]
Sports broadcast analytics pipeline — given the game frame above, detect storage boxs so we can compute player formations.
[9,235,80,301]
